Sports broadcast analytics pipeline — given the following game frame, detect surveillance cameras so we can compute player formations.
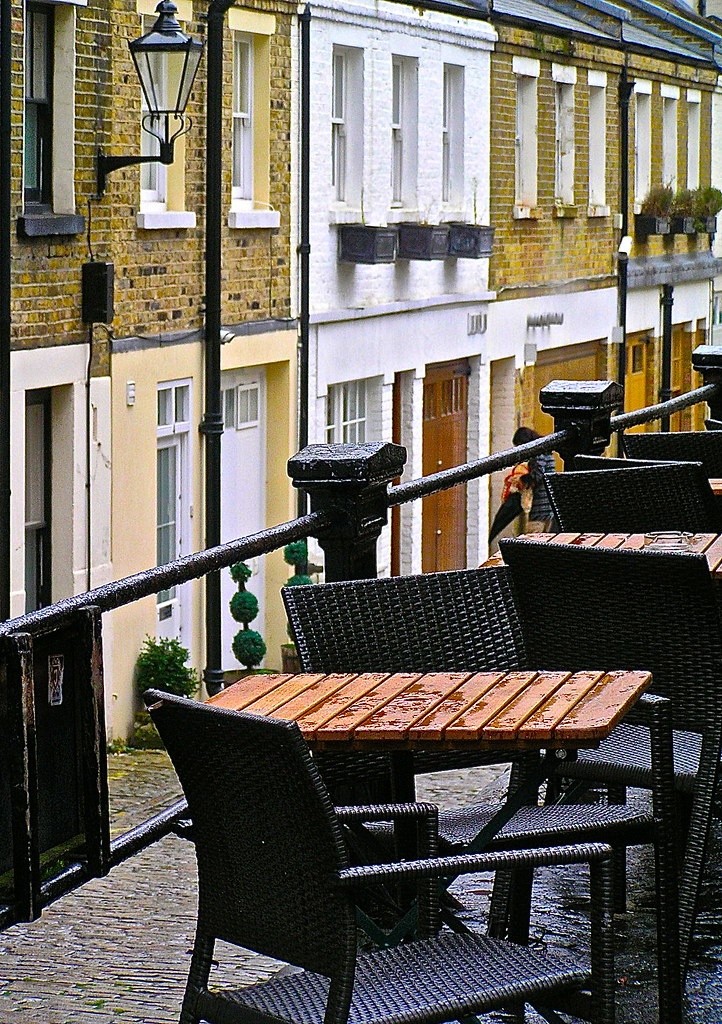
[220,329,235,344]
[619,235,634,260]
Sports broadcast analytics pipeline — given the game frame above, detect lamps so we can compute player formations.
[96,0,203,194]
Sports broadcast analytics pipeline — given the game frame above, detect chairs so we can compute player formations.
[621,430,721,508]
[142,687,615,1024]
[541,461,722,537]
[279,563,650,964]
[491,537,722,997]
[574,453,676,471]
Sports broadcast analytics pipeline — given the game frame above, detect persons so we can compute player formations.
[508,427,556,534]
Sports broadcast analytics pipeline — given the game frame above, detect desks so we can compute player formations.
[203,668,652,866]
[706,478,722,496]
[512,531,722,579]
